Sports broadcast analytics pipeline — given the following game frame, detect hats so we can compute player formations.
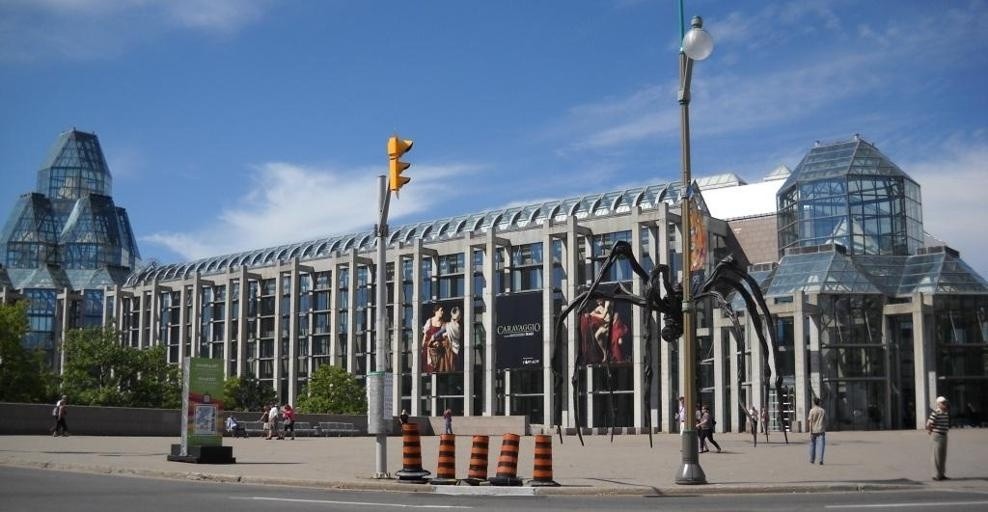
[936,396,950,403]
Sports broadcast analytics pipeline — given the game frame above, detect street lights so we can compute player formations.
[677,0,714,484]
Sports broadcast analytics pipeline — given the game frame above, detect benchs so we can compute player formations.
[224,420,361,438]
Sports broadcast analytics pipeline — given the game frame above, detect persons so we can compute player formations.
[678,397,685,452]
[807,398,829,465]
[749,407,760,434]
[759,408,769,434]
[421,305,461,373]
[399,409,408,425]
[258,404,295,440]
[696,405,721,453]
[226,413,248,437]
[866,402,883,430]
[927,396,950,481]
[695,403,709,452]
[48,394,72,437]
[443,409,452,435]
[580,298,629,363]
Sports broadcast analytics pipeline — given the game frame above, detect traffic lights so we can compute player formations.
[387,136,413,191]
